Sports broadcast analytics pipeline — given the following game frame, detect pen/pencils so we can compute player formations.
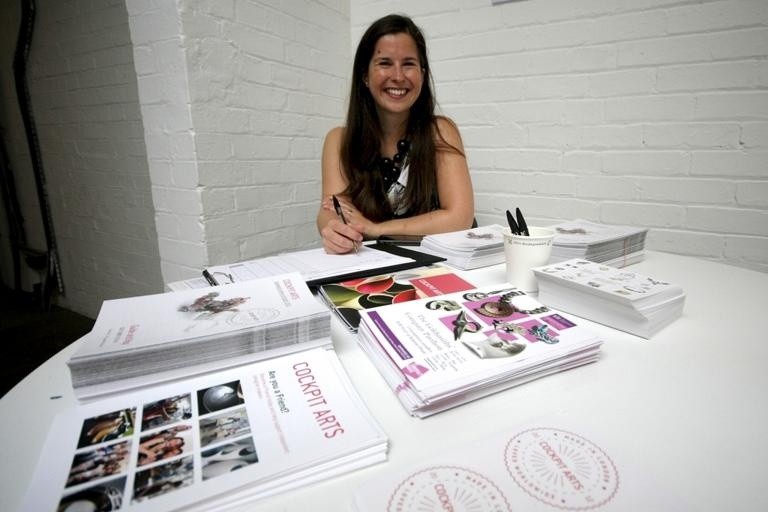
[333,195,358,255]
[203,269,217,286]
[506,207,530,236]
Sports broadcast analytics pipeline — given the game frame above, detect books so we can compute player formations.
[420,223,506,271]
[25,345,389,511]
[547,219,649,268]
[316,264,476,334]
[356,282,605,419]
[67,272,331,402]
[532,258,685,339]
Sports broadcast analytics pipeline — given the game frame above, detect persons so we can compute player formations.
[313,14,477,255]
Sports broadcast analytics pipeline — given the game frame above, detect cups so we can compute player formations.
[502,226,555,292]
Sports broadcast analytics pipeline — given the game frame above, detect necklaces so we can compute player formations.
[383,135,409,194]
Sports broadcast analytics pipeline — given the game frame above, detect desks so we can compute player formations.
[0,248,765,511]
